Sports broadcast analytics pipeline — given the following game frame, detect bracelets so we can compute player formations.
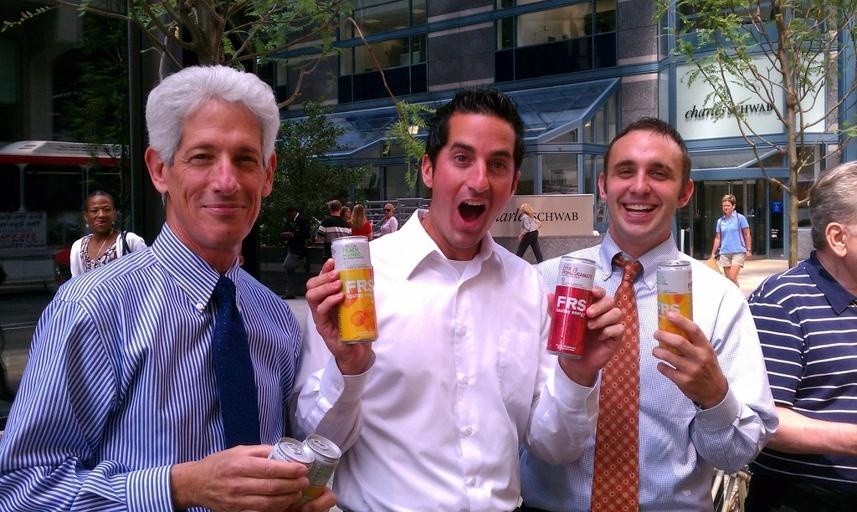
[747,248,751,252]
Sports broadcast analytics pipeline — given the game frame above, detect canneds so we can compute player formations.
[331,236,379,345]
[547,256,596,361]
[657,261,692,355]
[268,434,343,512]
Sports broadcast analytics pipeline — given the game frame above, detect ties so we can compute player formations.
[212,280,263,449]
[590,252,643,512]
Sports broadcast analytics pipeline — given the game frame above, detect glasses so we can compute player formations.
[383,208,391,212]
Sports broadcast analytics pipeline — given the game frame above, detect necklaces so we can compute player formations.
[91,227,114,257]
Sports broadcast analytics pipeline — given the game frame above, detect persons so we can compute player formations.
[741,161,857,511]
[285,86,623,511]
[515,201,544,265]
[281,199,400,301]
[711,193,752,287]
[67,188,150,279]
[1,65,338,511]
[518,116,778,511]
